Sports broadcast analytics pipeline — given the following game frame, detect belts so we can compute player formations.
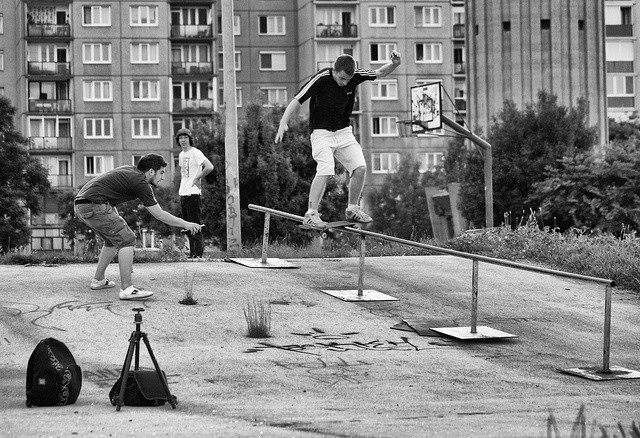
[75,199,106,205]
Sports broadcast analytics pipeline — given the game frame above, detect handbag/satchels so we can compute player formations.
[109,370,167,406]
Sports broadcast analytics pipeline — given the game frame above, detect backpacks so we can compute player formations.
[26,338,82,408]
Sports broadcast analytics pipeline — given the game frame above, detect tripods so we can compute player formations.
[114,307,179,412]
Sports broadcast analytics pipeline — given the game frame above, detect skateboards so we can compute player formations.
[295,220,376,238]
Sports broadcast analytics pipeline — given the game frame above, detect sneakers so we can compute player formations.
[303,211,328,229]
[91,278,115,289]
[119,285,154,300]
[346,205,373,223]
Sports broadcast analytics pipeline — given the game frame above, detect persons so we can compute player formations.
[72,155,202,300]
[273,50,401,228]
[176,128,214,261]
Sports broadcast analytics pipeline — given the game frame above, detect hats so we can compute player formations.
[176,128,193,140]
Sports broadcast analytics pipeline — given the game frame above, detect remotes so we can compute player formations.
[180,224,206,235]
